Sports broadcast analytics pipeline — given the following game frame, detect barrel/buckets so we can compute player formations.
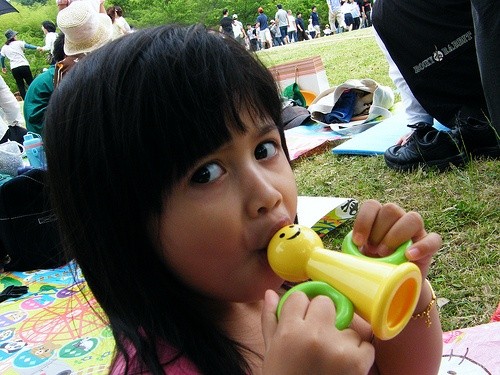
[0,141,25,176]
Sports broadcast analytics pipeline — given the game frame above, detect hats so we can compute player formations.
[325,24,329,27]
[5,28,18,40]
[258,7,263,13]
[56,4,112,57]
[233,14,237,18]
[281,106,312,130]
[270,20,275,23]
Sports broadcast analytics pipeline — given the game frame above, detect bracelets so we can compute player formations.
[409,276,437,328]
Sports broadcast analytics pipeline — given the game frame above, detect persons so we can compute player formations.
[41,24,442,375]
[219,0,376,50]
[0,0,135,145]
[371,0,500,172]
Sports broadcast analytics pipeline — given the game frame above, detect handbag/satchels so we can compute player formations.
[47,53,54,65]
[0,169,75,271]
[308,78,395,135]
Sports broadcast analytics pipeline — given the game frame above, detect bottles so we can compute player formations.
[23,134,47,168]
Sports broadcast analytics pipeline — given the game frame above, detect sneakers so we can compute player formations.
[384,122,467,172]
[451,111,500,159]
